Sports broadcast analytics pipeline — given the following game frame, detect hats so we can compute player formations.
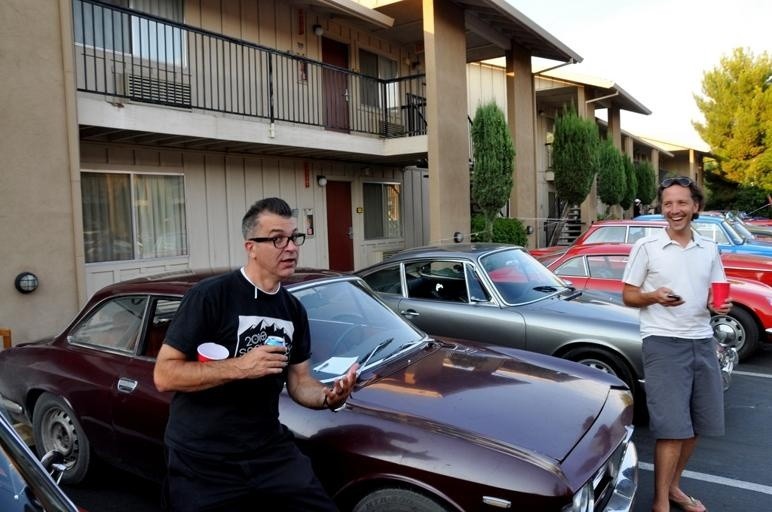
[635,199,641,202]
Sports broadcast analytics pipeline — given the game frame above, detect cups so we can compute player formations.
[710,282,729,311]
[197,341,229,363]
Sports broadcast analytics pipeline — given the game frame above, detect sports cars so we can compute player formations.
[358,242,739,428]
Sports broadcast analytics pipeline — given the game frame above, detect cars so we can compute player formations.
[0,266,641,512]
[0,405,90,512]
[81,216,185,264]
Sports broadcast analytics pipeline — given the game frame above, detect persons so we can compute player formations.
[622,175,733,512]
[152,198,360,512]
[633,199,641,217]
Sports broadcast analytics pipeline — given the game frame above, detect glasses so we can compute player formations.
[662,178,691,188]
[248,233,305,249]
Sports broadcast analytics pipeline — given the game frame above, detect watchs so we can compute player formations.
[325,390,347,413]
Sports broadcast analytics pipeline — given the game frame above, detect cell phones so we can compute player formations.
[668,294,681,301]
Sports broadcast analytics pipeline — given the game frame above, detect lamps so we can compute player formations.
[312,23,326,37]
[526,223,535,235]
[313,173,329,187]
[12,268,42,298]
[454,231,464,244]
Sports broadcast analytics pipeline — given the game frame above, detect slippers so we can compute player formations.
[670,492,708,512]
